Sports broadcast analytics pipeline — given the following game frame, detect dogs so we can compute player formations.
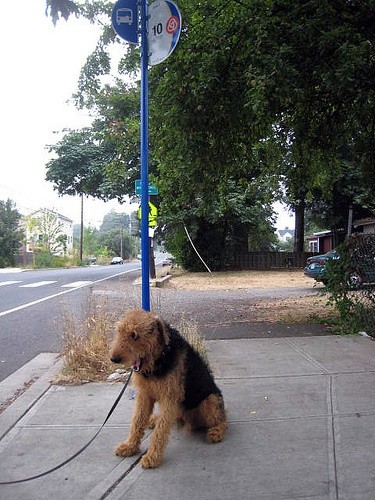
[109,308,228,470]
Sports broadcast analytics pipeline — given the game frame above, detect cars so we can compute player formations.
[110,257,123,265]
[304,233,375,288]
